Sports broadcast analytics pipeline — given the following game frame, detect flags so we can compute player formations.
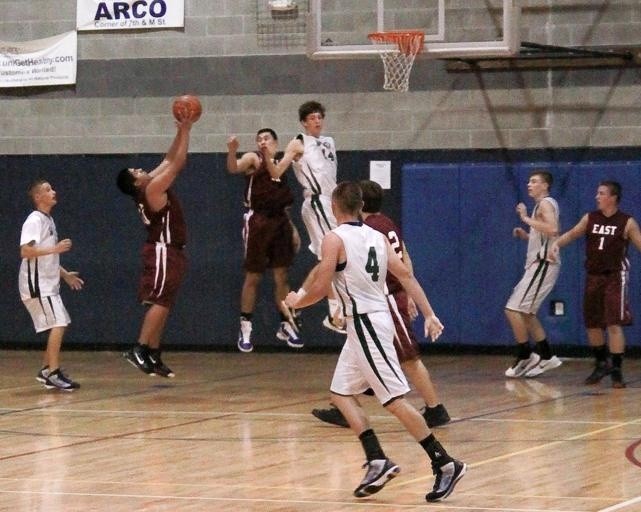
[2,28,83,94]
[76,0,191,35]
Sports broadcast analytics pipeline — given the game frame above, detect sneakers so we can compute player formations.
[147,348,176,379]
[280,299,304,334]
[425,455,468,503]
[311,401,352,429]
[35,365,51,383]
[353,457,402,497]
[525,354,563,379]
[276,321,305,349]
[420,403,451,429]
[504,351,542,379]
[237,316,254,353]
[584,364,613,386]
[43,368,81,392]
[122,341,158,378]
[610,365,627,389]
[322,315,348,335]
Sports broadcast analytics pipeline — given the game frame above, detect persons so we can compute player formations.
[116,108,202,379]
[225,126,308,354]
[15,177,86,392]
[548,180,641,390]
[502,170,565,381]
[260,101,352,336]
[284,181,470,504]
[311,181,453,431]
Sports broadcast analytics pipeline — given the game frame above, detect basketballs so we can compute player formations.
[173,95,202,123]
